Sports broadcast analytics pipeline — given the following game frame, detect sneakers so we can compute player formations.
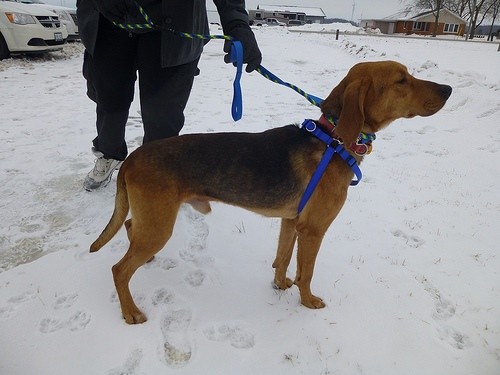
[83,147,126,192]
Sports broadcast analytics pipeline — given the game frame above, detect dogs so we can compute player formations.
[89,61,453,324]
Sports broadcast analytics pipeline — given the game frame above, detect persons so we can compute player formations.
[75,0,262,192]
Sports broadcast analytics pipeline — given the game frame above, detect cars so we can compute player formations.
[256,18,286,27]
[287,20,306,27]
[0,0,69,61]
[16,0,82,40]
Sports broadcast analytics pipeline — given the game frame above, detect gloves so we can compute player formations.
[224,22,262,73]
[91,0,133,21]
